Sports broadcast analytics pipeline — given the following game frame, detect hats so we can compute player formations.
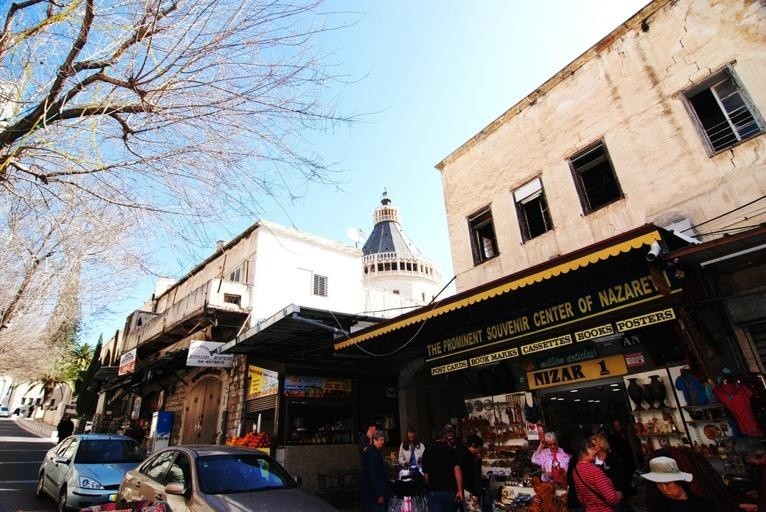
[443,424,455,433]
[640,456,693,485]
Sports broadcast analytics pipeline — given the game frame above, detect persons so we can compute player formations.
[640,455,708,512]
[358,425,392,512]
[422,424,494,512]
[12,406,21,416]
[56,414,73,444]
[27,404,34,418]
[531,433,572,486]
[566,432,622,512]
[398,426,425,468]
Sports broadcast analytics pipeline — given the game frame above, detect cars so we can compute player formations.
[0,406,11,417]
[117,441,340,512]
[34,432,149,512]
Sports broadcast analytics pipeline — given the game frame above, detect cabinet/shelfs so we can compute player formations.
[623,361,738,459]
